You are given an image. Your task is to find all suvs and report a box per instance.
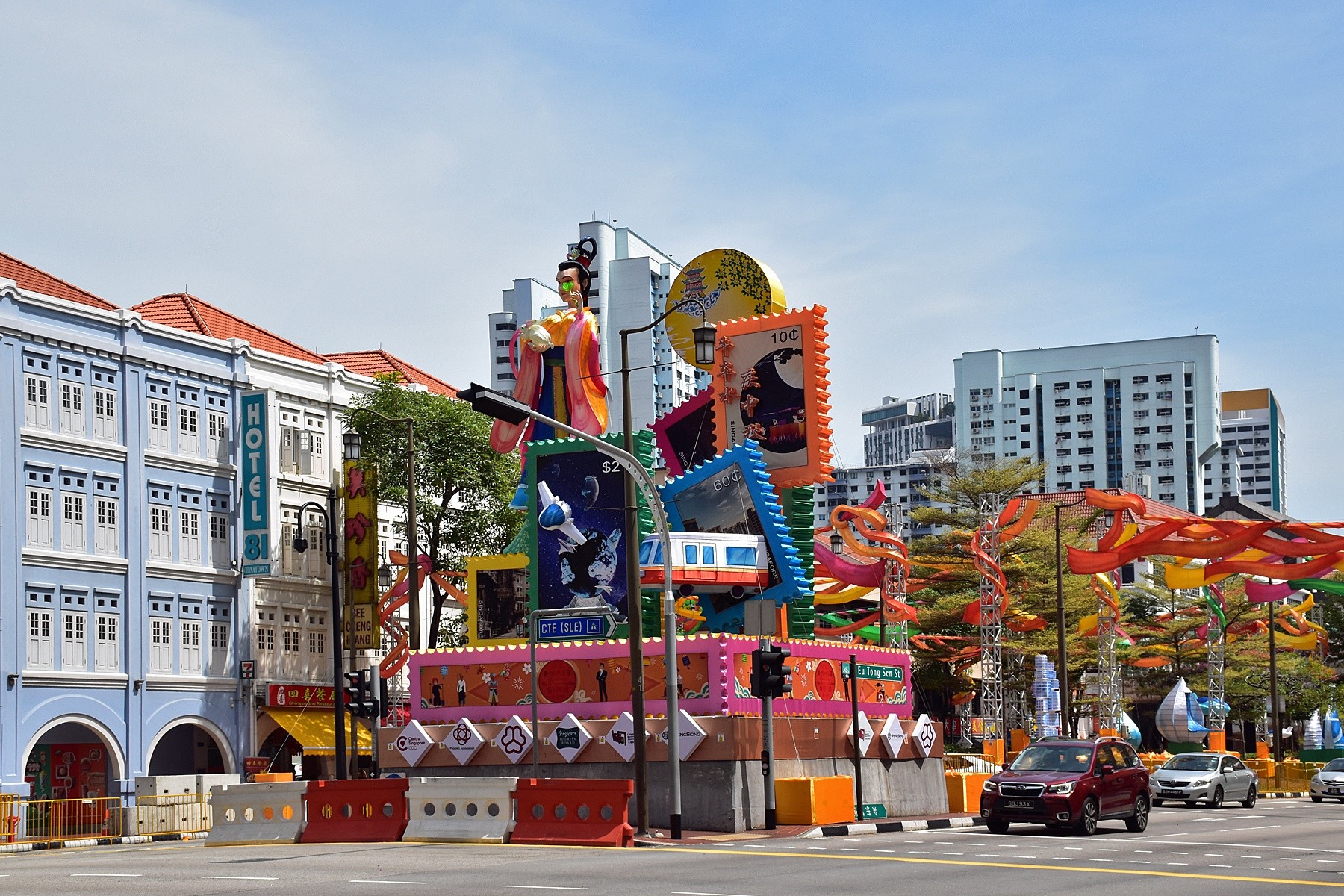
[979,734,1152,837]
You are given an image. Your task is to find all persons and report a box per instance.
[491,237,608,509]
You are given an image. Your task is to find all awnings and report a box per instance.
[380,705,411,727]
[259,706,373,756]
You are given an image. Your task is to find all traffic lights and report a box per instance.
[751,648,785,697]
[344,669,366,718]
[771,644,792,700]
[359,699,379,720]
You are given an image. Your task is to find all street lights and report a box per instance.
[1054,489,1115,740]
[617,298,719,835]
[292,500,348,780]
[456,381,684,840]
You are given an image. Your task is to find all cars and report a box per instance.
[1149,751,1259,809]
[942,752,1004,776]
[1309,758,1344,805]
